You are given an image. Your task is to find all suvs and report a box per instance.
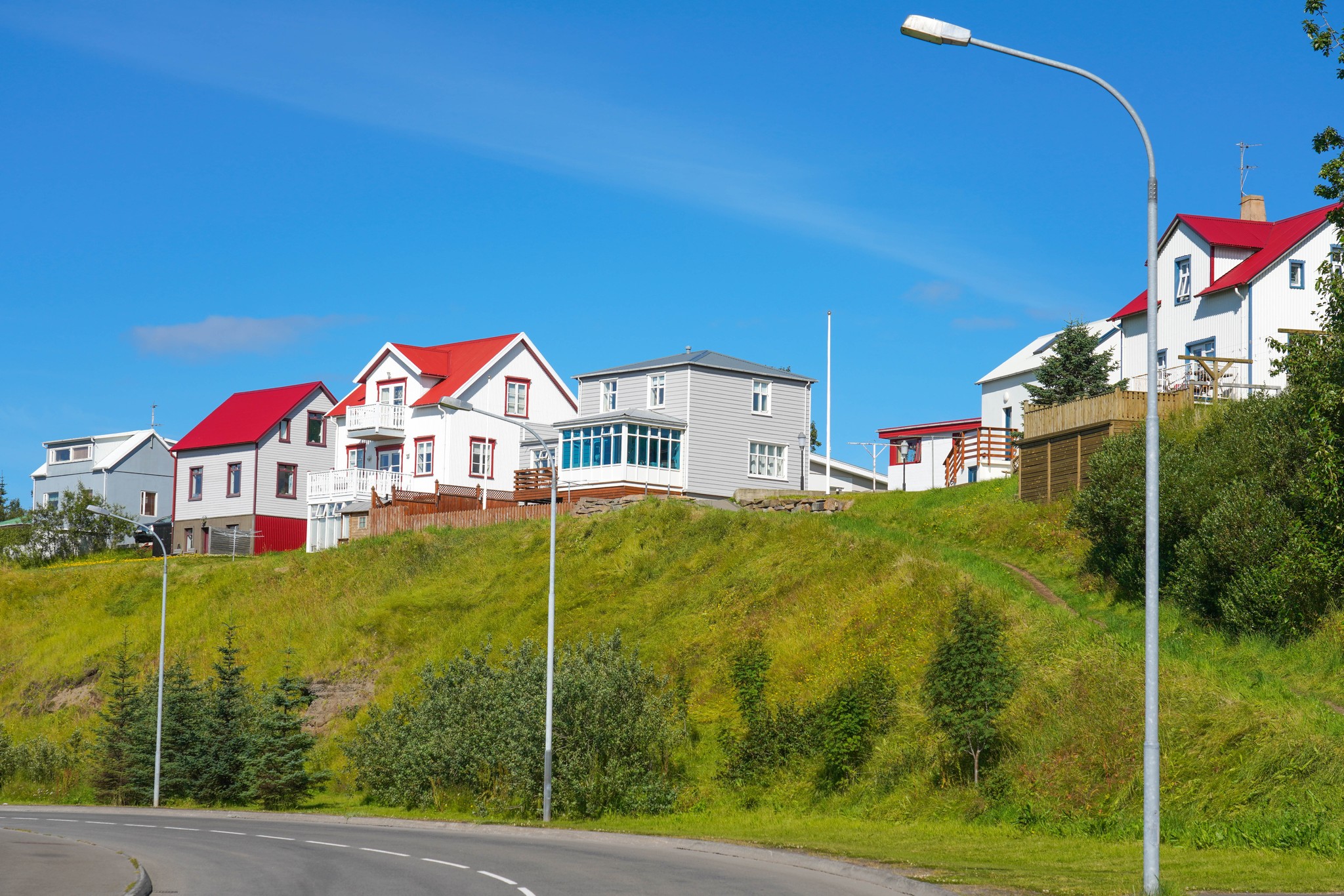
[133,514,173,543]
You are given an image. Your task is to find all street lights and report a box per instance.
[900,440,909,492]
[439,395,557,824]
[85,503,168,809]
[899,11,1160,896]
[797,432,806,490]
